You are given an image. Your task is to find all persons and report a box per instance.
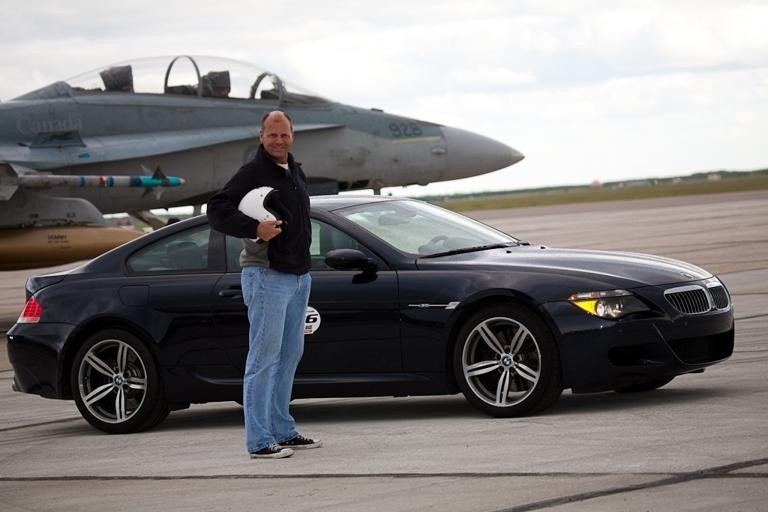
[207,111,323,458]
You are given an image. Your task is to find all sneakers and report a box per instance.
[277,433,325,449]
[249,440,294,459]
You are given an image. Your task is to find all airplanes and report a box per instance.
[0,49,535,276]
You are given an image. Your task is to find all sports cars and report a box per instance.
[2,193,738,441]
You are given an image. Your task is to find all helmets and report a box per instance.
[235,185,285,244]
